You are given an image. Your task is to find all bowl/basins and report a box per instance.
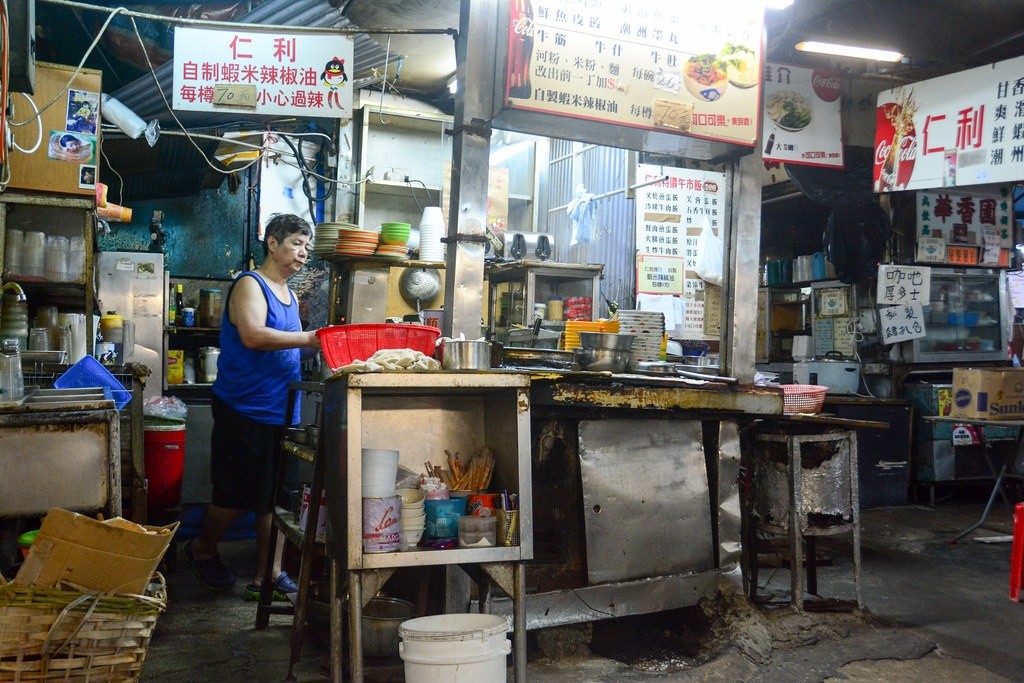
[634,361,679,375]
[380,207,447,263]
[766,91,813,133]
[396,486,426,546]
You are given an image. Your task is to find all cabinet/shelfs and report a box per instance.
[755,287,810,361]
[488,262,605,338]
[7,60,105,195]
[316,368,538,683]
[355,101,547,257]
[912,265,1007,365]
[743,427,866,612]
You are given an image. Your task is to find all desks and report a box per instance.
[919,414,1024,547]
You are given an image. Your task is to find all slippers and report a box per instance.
[181,537,235,590]
[244,570,298,601]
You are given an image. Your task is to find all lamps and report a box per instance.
[796,35,903,73]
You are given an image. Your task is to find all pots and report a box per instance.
[440,340,492,371]
[573,331,638,372]
[340,595,418,657]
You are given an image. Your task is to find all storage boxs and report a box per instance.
[814,319,833,359]
[832,318,853,356]
[820,287,849,317]
[948,365,1024,418]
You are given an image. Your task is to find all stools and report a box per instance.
[1008,501,1024,602]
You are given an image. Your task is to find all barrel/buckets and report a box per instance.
[142,424,187,509]
[360,495,402,554]
[398,612,512,683]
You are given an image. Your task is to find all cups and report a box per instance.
[101,314,135,365]
[180,307,196,326]
[425,488,502,547]
[0,230,85,282]
[198,288,222,328]
[71,322,98,363]
[28,328,48,352]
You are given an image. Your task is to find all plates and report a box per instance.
[565,308,667,364]
[313,222,410,257]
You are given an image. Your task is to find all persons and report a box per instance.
[71,100,90,131]
[180,214,322,601]
[84,170,93,184]
[60,135,80,153]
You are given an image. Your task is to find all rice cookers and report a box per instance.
[793,349,860,396]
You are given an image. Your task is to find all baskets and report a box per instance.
[315,323,441,370]
[56,354,132,411]
[778,385,830,413]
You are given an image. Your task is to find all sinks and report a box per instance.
[32,385,111,395]
[24,396,116,411]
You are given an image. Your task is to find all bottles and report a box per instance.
[764,130,776,155]
[878,131,903,192]
[168,282,184,325]
[199,346,220,382]
[0,337,24,402]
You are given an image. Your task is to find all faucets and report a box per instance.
[0,282,27,304]
